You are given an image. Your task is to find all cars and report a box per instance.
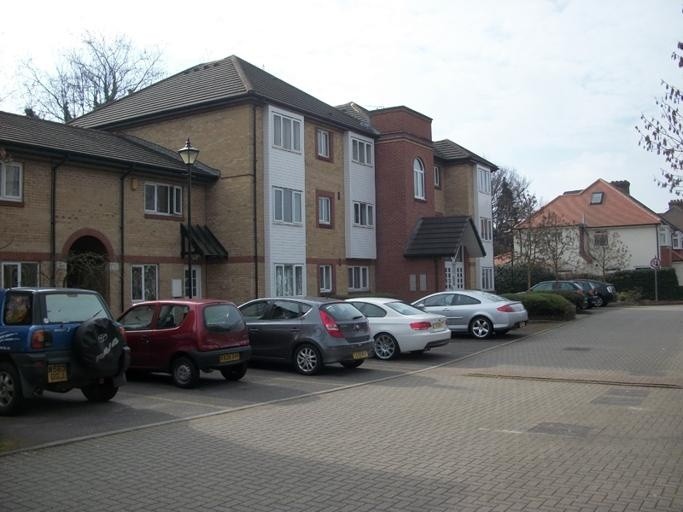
[516,281,598,313]
[335,294,456,368]
[224,294,376,379]
[405,288,530,342]
[105,295,257,393]
[567,278,617,308]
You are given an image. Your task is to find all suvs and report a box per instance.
[0,282,133,417]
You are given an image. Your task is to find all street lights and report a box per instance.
[176,135,201,302]
